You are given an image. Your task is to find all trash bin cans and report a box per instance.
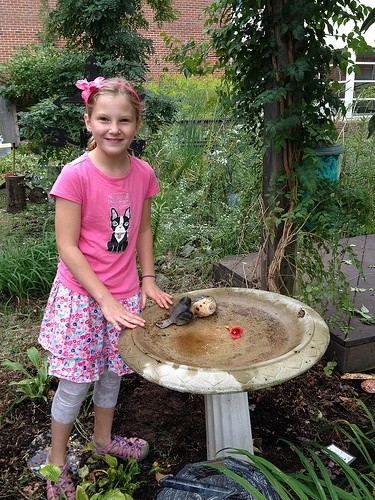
[302,140,344,233]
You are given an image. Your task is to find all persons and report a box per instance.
[36,75,174,498]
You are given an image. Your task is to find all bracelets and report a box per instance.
[142,275,156,280]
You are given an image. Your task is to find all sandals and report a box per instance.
[90,436,148,462]
[44,462,76,500]
